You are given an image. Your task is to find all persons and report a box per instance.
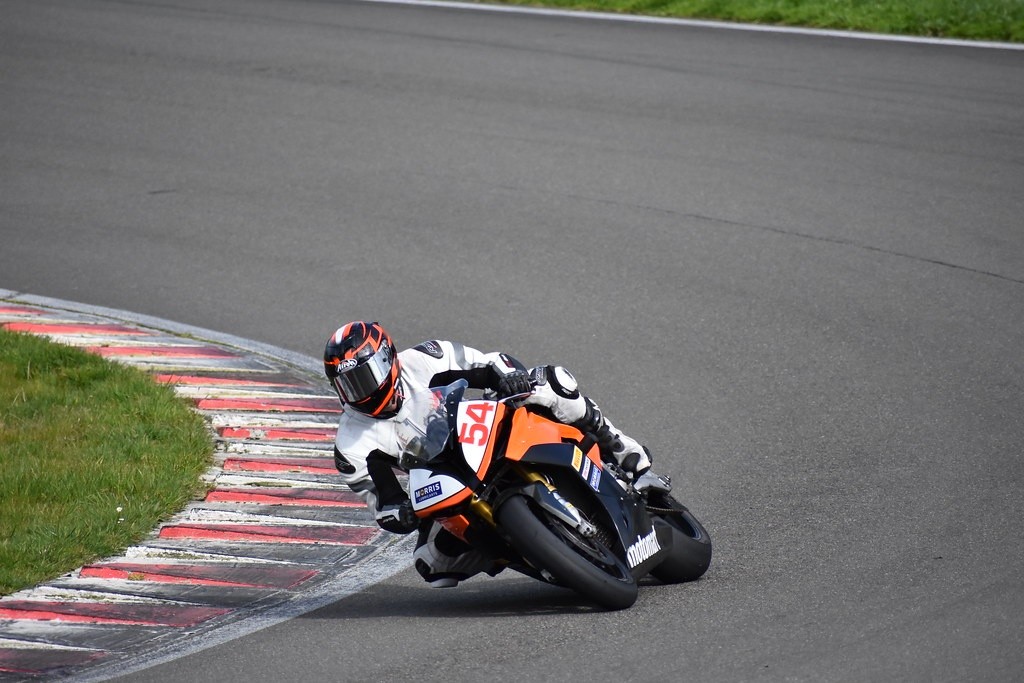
[325,321,652,589]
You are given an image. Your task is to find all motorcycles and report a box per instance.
[393,378,713,611]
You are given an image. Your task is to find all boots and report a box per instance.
[571,397,651,474]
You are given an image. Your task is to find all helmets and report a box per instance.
[323,320,401,417]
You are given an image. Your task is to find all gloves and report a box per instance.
[499,369,532,401]
[398,500,422,531]
